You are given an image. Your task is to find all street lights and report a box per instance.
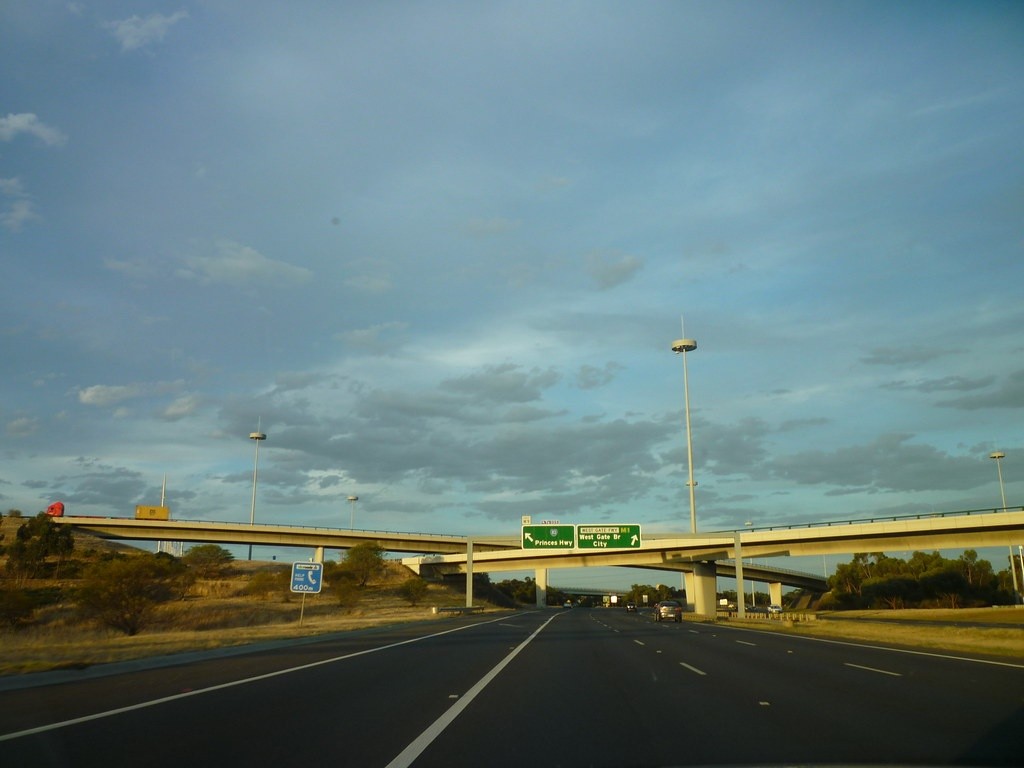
[989,447,1018,593]
[247,416,267,561]
[671,315,698,534]
[744,520,756,607]
[348,494,358,528]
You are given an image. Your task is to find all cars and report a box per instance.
[653,600,682,623]
[627,602,638,613]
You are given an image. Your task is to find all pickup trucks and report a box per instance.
[767,604,783,614]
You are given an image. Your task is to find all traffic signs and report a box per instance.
[575,524,642,548]
[521,524,576,551]
